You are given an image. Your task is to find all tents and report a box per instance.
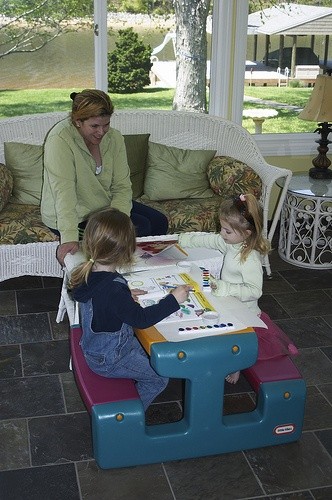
[245,2,332,76]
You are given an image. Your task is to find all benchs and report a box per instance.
[0,109,293,284]
[224,311,306,453]
[69,324,185,469]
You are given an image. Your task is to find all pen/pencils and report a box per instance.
[164,285,196,293]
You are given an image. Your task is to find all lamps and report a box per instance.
[297,74,332,179]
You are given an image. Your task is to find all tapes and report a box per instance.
[202,311,220,325]
[176,261,193,272]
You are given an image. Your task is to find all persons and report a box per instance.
[39,88,169,268]
[67,208,191,416]
[176,193,275,384]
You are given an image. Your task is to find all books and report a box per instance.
[127,271,217,325]
[131,240,179,259]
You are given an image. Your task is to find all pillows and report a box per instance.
[123,133,150,201]
[207,156,263,200]
[143,140,217,201]
[3,141,43,205]
[0,163,14,211]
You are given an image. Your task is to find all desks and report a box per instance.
[120,270,259,432]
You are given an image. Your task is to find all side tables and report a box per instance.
[275,170,332,270]
